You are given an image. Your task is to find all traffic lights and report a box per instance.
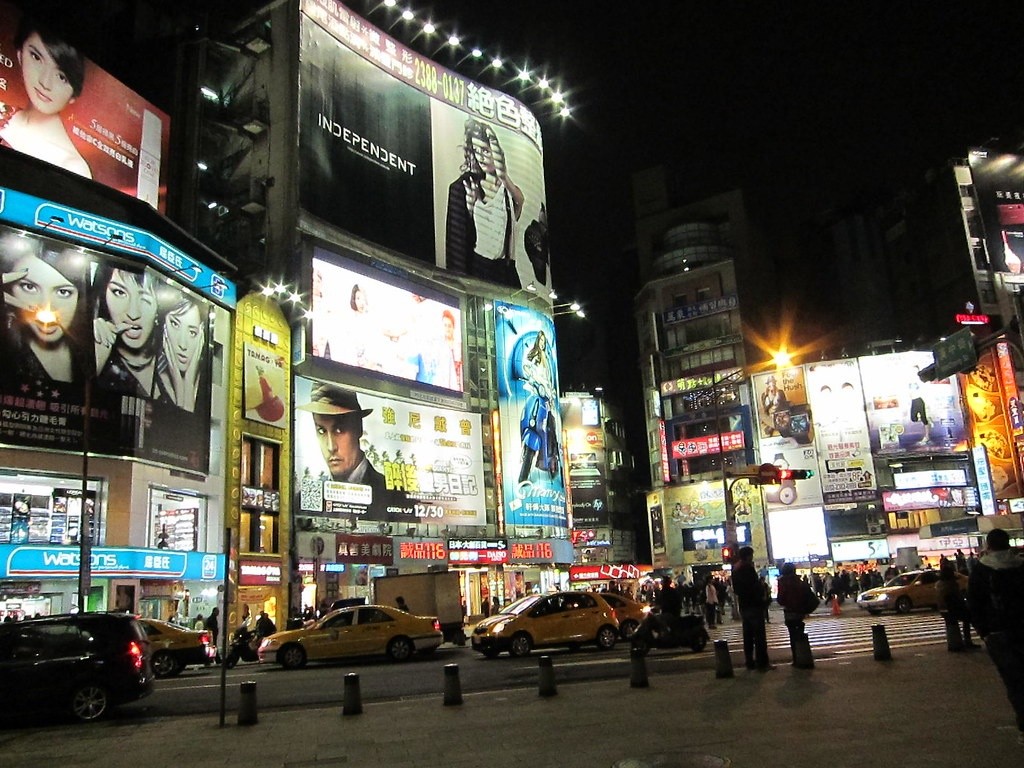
[722,545,735,564]
[781,468,813,480]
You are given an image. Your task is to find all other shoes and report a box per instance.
[708,625,717,629]
[1016,732,1024,744]
[745,660,773,672]
[964,639,982,649]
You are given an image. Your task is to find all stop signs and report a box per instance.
[758,462,777,484]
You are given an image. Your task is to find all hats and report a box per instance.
[295,382,373,418]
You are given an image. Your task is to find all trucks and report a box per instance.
[332,570,464,642]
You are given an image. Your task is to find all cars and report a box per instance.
[562,593,651,639]
[856,568,968,616]
[139,617,218,678]
[257,596,444,667]
[472,590,623,656]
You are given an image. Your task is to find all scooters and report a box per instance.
[224,626,260,668]
[518,365,560,482]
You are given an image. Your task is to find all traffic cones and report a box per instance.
[831,598,842,616]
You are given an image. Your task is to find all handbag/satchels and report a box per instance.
[802,583,820,614]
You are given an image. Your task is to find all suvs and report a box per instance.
[0,613,154,728]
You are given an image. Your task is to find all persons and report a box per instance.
[967,528,1024,732]
[195,603,330,665]
[153,281,210,415]
[0,11,92,180]
[731,546,770,671]
[908,364,935,446]
[445,115,525,289]
[914,548,982,652]
[294,381,421,523]
[761,375,786,435]
[461,596,500,627]
[89,263,158,397]
[312,266,464,392]
[526,330,557,473]
[0,227,89,405]
[396,596,409,613]
[776,562,815,669]
[592,565,900,630]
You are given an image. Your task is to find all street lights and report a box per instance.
[36,310,86,612]
[713,351,790,566]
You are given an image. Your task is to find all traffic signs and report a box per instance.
[931,325,979,381]
